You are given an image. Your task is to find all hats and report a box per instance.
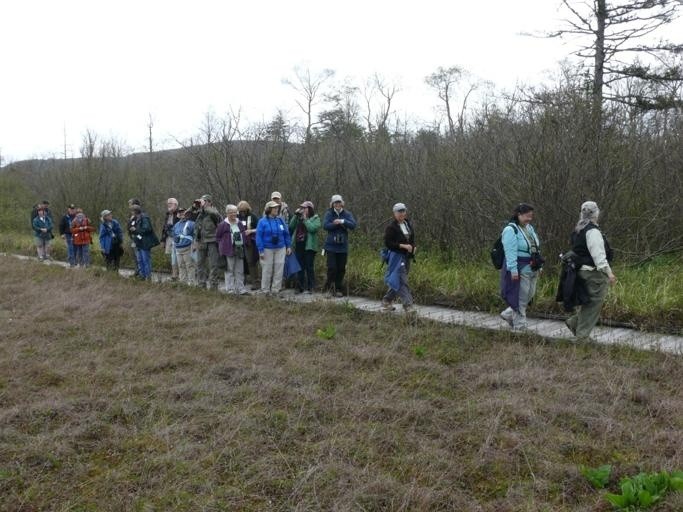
[393,203,406,211]
[101,210,112,216]
[330,195,344,207]
[201,194,211,202]
[300,201,313,208]
[265,192,281,208]
[581,201,597,214]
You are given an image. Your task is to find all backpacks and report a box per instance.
[491,224,518,269]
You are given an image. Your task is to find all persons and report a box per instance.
[380,202,418,315]
[500,203,546,332]
[320,195,356,300]
[556,200,617,343]
[31,200,96,269]
[128,191,322,296]
[97,210,124,275]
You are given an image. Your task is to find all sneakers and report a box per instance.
[403,305,417,313]
[228,289,247,295]
[383,303,395,311]
[500,311,529,332]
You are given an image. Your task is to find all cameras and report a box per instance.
[531,253,545,272]
[194,199,201,207]
[272,236,281,246]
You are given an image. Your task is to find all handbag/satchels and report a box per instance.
[137,217,160,249]
[381,247,390,263]
[285,252,301,278]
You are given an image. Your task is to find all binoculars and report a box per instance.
[337,234,344,244]
[193,200,202,204]
[532,252,542,268]
[294,208,305,215]
[234,232,241,241]
[406,244,415,258]
